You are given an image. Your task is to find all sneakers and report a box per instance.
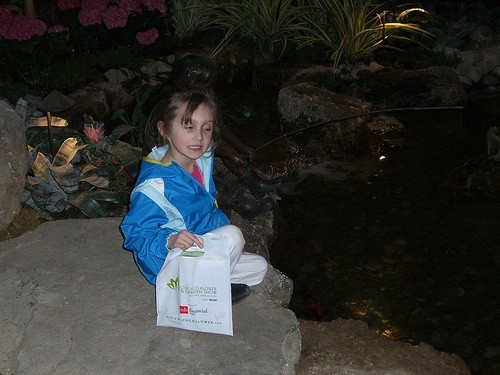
[229,283,250,305]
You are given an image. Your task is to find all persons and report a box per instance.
[119,82,269,308]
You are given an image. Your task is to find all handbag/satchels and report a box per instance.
[153,233,234,337]
